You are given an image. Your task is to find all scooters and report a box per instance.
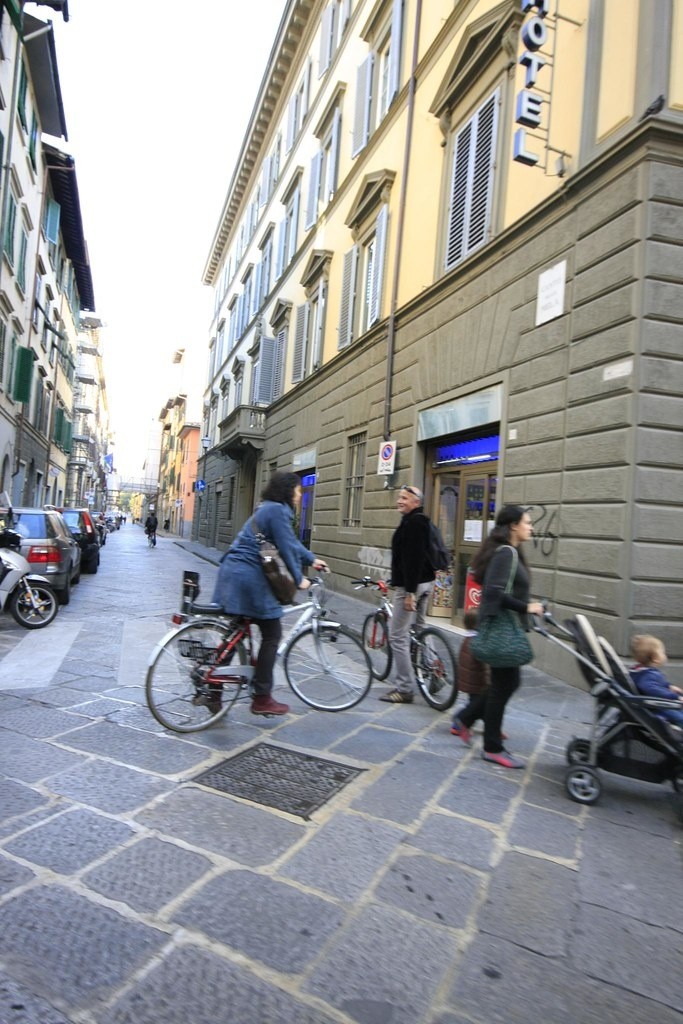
[0,490,59,630]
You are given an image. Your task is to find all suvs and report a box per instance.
[55,505,103,574]
[0,504,84,605]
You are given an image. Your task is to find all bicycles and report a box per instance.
[145,526,157,545]
[145,562,373,735]
[351,575,459,711]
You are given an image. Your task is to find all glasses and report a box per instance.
[401,485,420,498]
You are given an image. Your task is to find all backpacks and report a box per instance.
[409,512,452,572]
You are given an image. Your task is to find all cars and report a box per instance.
[89,510,124,545]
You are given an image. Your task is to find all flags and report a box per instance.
[104,454,113,472]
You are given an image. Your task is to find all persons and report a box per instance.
[200,470,332,717]
[453,496,538,774]
[143,511,157,546]
[382,486,452,704]
[627,628,682,736]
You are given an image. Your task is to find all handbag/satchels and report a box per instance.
[468,601,533,668]
[256,534,295,605]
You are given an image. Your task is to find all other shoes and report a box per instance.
[450,726,474,735]
[454,719,475,746]
[482,748,527,768]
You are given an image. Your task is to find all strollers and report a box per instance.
[527,597,682,805]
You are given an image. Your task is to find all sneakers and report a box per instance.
[206,690,222,713]
[426,672,445,695]
[251,692,289,715]
[380,689,414,703]
